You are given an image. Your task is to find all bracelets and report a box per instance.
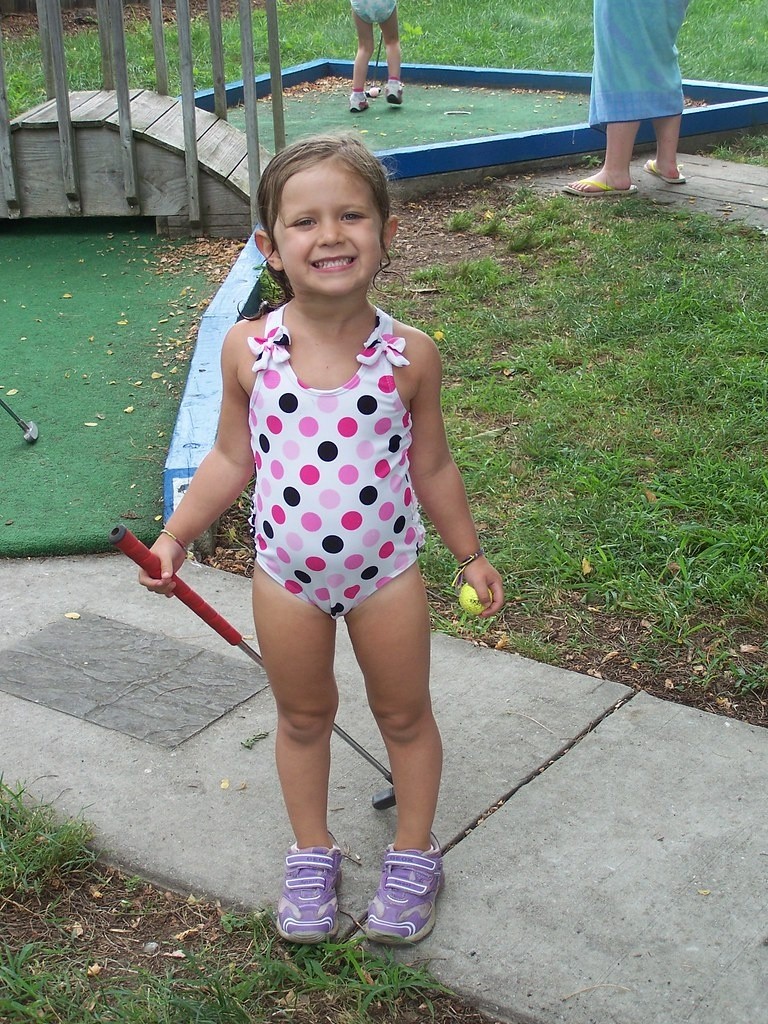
[451,547,484,588]
[160,530,188,558]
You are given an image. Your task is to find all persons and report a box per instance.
[564,0,691,196]
[350,0,403,111]
[137,133,506,947]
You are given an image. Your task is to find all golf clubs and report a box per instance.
[108,523,397,811]
[365,31,382,97]
[0,399,39,443]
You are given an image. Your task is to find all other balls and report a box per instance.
[369,88,378,97]
[457,583,493,615]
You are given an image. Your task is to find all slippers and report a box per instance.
[643,157,686,185]
[562,178,639,196]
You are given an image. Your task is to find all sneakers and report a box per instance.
[384,83,402,105]
[277,829,343,943]
[365,830,444,945]
[349,94,369,112]
